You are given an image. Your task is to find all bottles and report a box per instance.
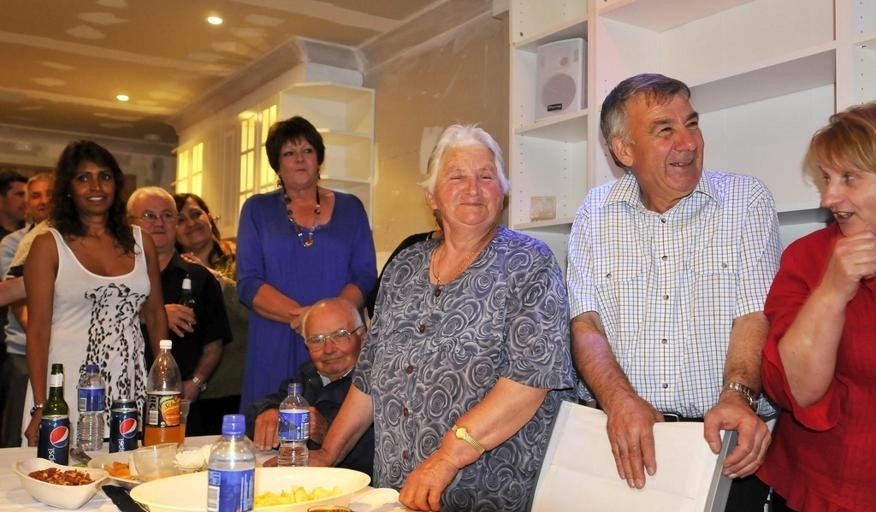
[277,383,310,467]
[205,414,255,512]
[144,340,181,449]
[76,365,104,451]
[177,272,195,330]
[42,364,68,415]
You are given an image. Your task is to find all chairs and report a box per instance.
[527,397,738,511]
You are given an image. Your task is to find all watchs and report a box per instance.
[720,382,761,411]
[450,422,488,458]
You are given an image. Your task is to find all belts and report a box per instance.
[662,414,704,422]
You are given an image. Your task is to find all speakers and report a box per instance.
[534,37,586,122]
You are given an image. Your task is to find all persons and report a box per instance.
[563,72,783,512]
[243,297,375,486]
[234,115,377,436]
[18,138,181,446]
[259,116,582,512]
[363,140,442,323]
[753,98,876,511]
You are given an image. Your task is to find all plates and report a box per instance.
[86,450,141,489]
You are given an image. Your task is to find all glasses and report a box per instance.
[129,212,177,222]
[302,327,361,348]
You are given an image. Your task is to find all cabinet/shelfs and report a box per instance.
[508,0,876,279]
[278,79,377,234]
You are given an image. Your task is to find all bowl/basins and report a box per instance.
[129,466,371,512]
[13,456,108,509]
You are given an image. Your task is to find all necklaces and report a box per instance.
[430,227,493,286]
[281,186,319,248]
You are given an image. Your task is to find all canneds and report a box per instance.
[109,408,137,453]
[38,414,69,465]
[111,399,137,408]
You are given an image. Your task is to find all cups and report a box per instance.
[132,442,176,482]
[529,195,556,221]
[177,398,190,443]
[306,505,352,512]
